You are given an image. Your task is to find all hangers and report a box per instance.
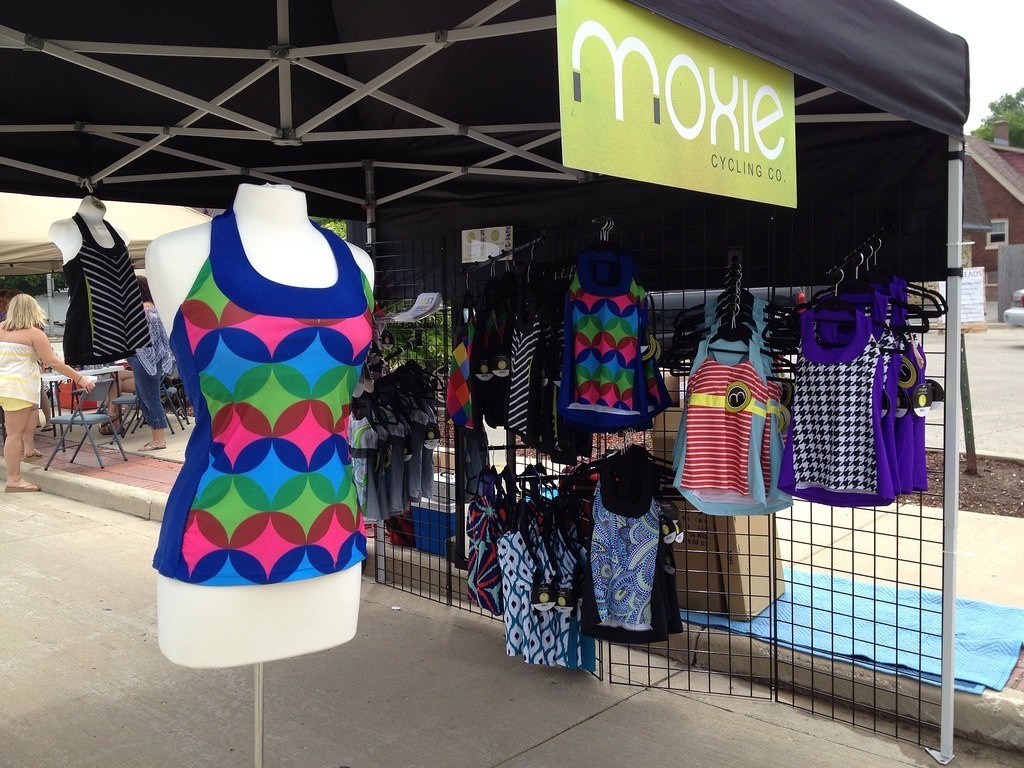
[658,232,949,383]
[451,427,687,576]
[352,340,443,427]
[445,212,614,292]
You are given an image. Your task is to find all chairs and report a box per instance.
[110,358,190,446]
[44,378,128,471]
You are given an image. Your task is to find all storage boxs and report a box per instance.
[411,498,456,555]
[58,376,97,409]
[650,368,785,621]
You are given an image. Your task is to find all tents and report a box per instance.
[0,192,216,276]
[0,0,973,764]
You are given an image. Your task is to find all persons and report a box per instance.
[98,362,178,436]
[145,183,376,670]
[49,195,152,364]
[0,288,97,493]
[126,275,174,452]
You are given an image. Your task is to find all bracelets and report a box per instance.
[77,376,82,383]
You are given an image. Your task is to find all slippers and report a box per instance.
[4,481,39,493]
[98,425,123,435]
[24,449,43,462]
[36,426,41,428]
[41,424,53,432]
[138,440,166,451]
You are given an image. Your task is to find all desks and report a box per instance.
[41,366,126,451]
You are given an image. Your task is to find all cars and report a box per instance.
[648,286,807,364]
[374,299,477,406]
